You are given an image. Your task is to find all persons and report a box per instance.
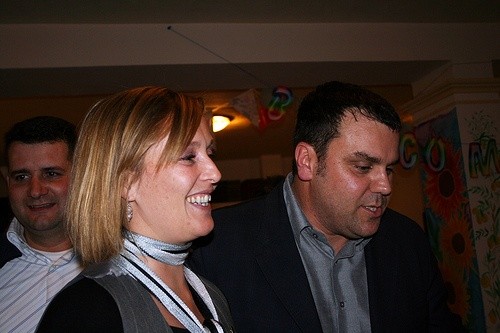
[34,84,238,333]
[0,114,87,333]
[183,79,471,333]
